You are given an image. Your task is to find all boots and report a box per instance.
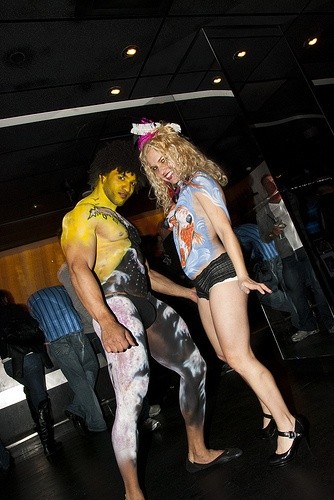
[35,399,63,456]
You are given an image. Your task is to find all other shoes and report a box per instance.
[64,409,86,438]
[223,364,234,373]
[186,447,242,473]
[143,418,162,431]
[291,328,320,342]
[149,404,162,417]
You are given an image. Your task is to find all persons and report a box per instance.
[257,174,334,342]
[0,290,55,455]
[130,118,310,470]
[233,224,317,342]
[60,142,243,500]
[57,262,107,362]
[27,286,107,431]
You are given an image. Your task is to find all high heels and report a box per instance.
[256,414,277,441]
[267,416,311,465]
